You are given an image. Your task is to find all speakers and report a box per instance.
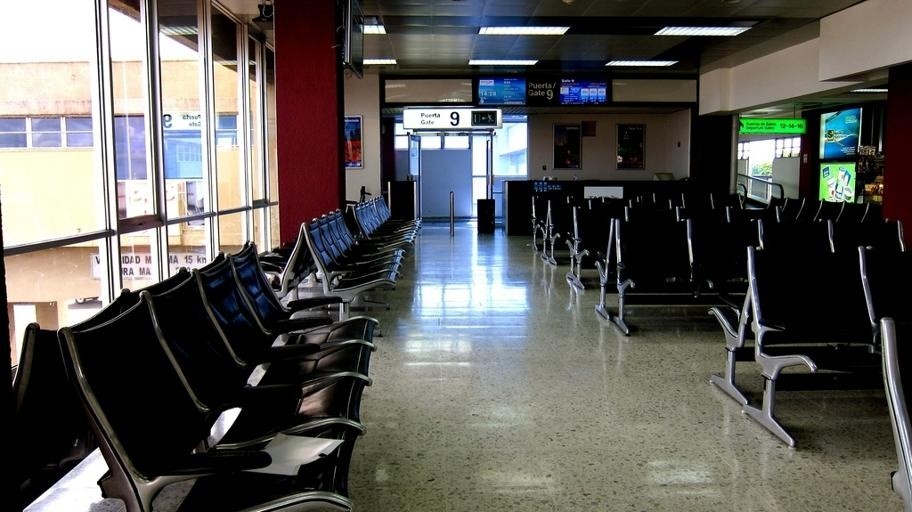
[477,199,495,234]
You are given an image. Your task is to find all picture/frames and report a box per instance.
[344,114,364,170]
[552,122,582,170]
[615,124,646,170]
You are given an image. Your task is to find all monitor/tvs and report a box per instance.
[344,0,364,80]
[471,76,527,108]
[557,75,613,107]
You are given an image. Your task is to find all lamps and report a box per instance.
[467,26,571,66]
[601,21,759,67]
[357,14,398,65]
